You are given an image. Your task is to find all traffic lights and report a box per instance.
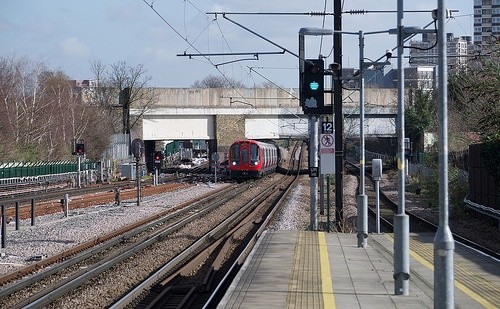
[304,58,324,113]
[76,143,84,155]
[154,151,161,168]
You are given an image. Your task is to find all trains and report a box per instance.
[228,139,278,182]
